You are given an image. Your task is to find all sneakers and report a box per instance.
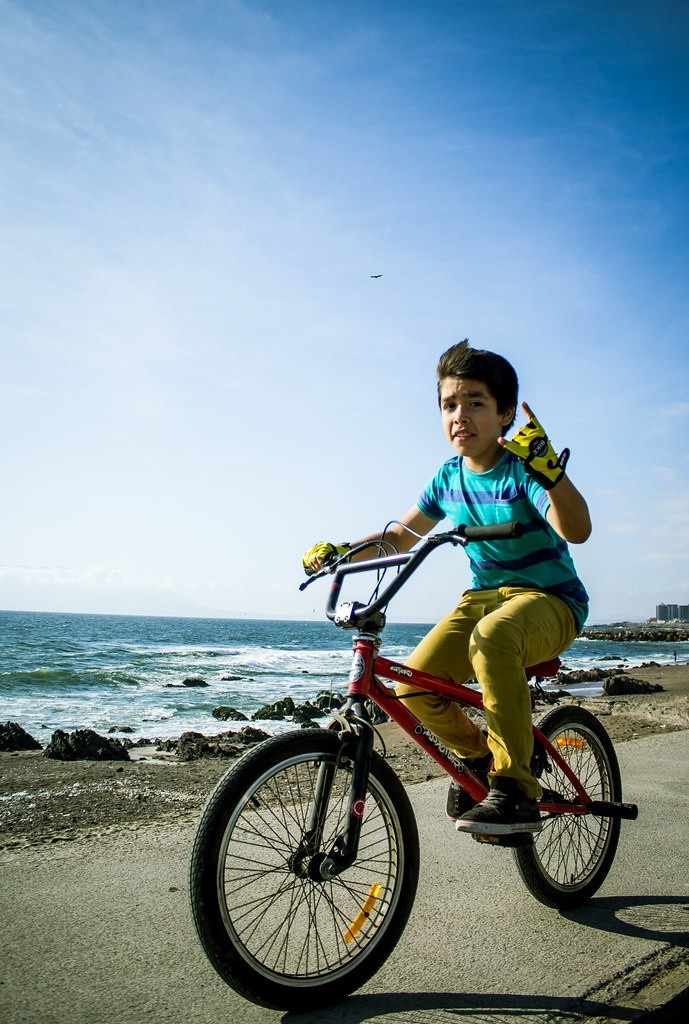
[444,729,494,822]
[456,777,542,834]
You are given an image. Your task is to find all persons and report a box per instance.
[299,340,592,829]
[671,649,677,663]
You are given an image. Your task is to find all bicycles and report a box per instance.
[186,516,640,1017]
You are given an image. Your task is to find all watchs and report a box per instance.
[337,541,353,563]
